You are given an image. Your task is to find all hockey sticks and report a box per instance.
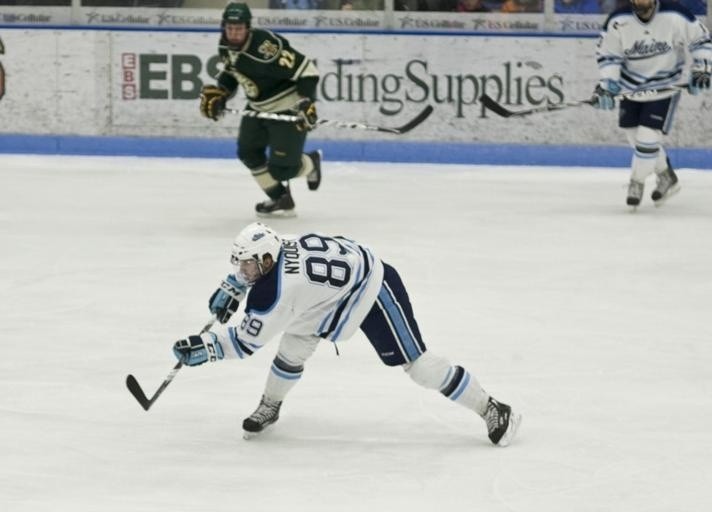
[223,105,433,134]
[479,85,689,118]
[127,314,219,411]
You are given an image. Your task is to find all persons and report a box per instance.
[175,223,512,444]
[588,0,691,204]
[673,1,712,94]
[200,3,324,214]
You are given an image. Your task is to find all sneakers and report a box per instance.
[650,166,679,200]
[307,150,321,191]
[242,392,283,432]
[255,189,295,214]
[479,395,512,445]
[625,178,644,206]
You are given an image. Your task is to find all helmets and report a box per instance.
[229,222,282,285]
[222,2,252,29]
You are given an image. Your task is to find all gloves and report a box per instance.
[171,326,245,367]
[199,85,227,120]
[590,78,623,111]
[687,70,712,96]
[294,98,318,133]
[208,273,248,325]
